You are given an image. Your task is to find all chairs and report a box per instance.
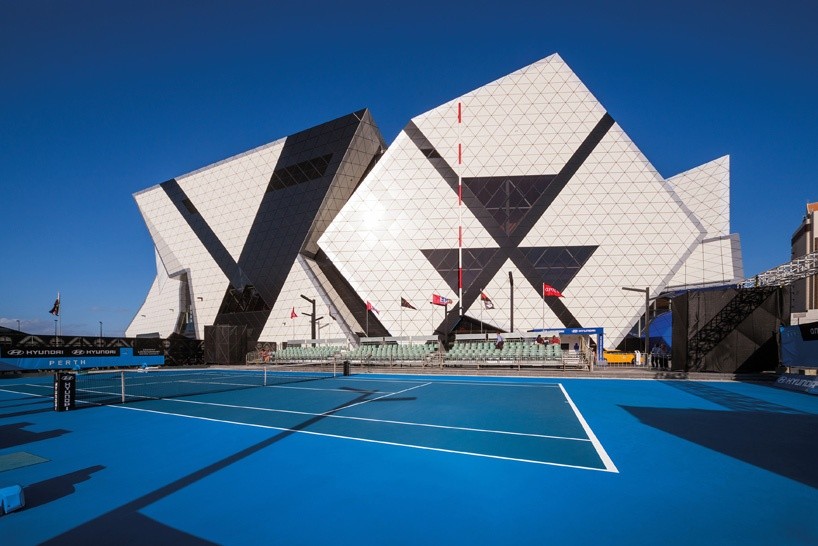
[272,339,564,360]
[562,359,583,369]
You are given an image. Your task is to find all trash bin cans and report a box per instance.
[343,361,350,376]
[53,372,76,412]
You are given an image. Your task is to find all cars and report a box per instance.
[604,350,645,364]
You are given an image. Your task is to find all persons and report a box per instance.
[535,335,579,353]
[650,344,669,368]
[494,332,503,350]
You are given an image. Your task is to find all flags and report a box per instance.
[401,297,418,311]
[481,292,494,310]
[543,283,565,297]
[367,301,379,315]
[433,295,453,306]
[290,308,298,319]
[50,300,59,315]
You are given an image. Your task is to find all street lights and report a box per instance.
[300,294,317,349]
[509,270,514,332]
[16,319,21,332]
[623,286,652,365]
[99,321,105,337]
